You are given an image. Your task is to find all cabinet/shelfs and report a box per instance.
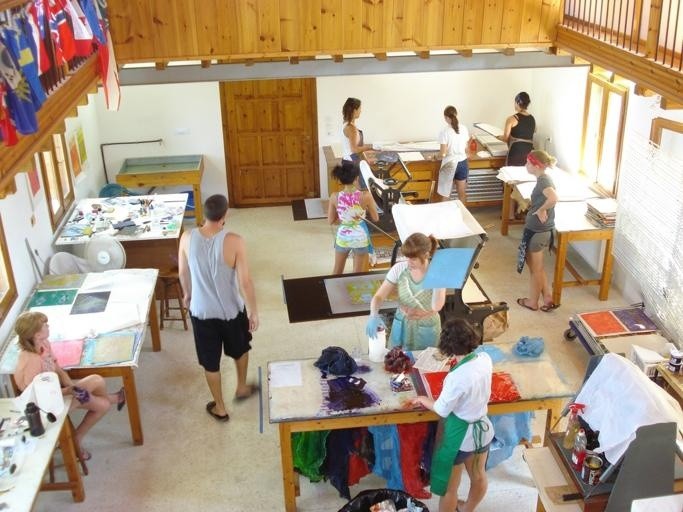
[534,431,683,512]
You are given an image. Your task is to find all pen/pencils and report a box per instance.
[163,200,184,202]
[139,198,153,215]
[8,428,29,436]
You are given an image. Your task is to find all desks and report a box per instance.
[265,348,574,512]
[321,139,508,208]
[500,171,616,306]
[652,358,683,405]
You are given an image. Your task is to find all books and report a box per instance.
[584,197,618,230]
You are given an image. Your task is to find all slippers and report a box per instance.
[57,442,91,460]
[541,301,561,311]
[117,389,127,411]
[236,383,259,400]
[517,297,537,311]
[207,401,229,423]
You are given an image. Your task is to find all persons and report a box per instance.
[432,106,470,209]
[176,193,260,424]
[340,97,375,193]
[406,316,494,510]
[516,148,560,313]
[364,232,448,350]
[326,160,380,278]
[494,92,537,221]
[12,311,127,462]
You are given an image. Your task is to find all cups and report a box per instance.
[580,455,604,485]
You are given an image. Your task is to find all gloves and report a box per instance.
[365,317,387,340]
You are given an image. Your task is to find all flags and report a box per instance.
[0,0,124,149]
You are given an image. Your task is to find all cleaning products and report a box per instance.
[470,135,477,151]
[563,403,585,449]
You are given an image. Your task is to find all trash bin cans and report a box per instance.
[336,488,430,512]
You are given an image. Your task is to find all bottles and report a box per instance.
[585,430,601,458]
[668,350,683,374]
[368,328,386,363]
[24,401,57,436]
